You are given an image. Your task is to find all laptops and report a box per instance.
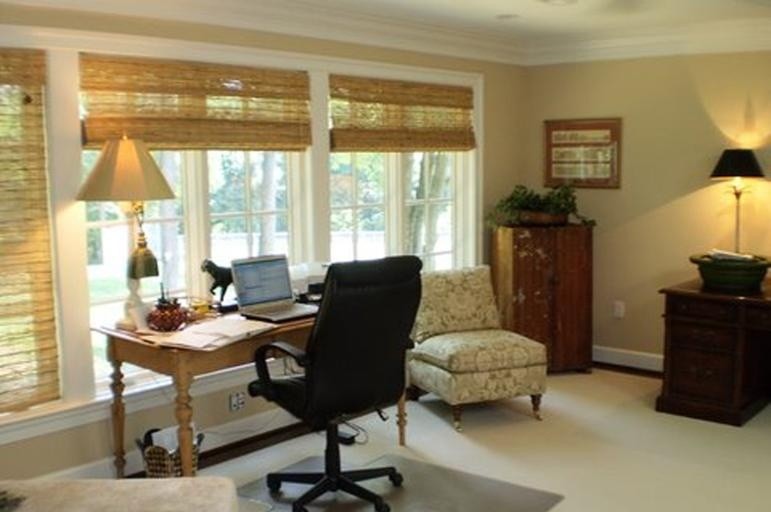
[231,254,319,324]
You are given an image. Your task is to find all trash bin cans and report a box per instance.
[136,428,205,478]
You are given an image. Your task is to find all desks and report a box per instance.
[90,298,407,478]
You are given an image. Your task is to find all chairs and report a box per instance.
[249,256,423,511]
[405,263,549,432]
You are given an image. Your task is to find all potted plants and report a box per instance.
[495,183,577,224]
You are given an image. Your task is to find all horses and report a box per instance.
[200,259,235,304]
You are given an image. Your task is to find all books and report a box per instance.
[707,247,759,262]
[128,301,279,353]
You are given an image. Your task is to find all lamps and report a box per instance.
[76,128,176,331]
[708,148,764,254]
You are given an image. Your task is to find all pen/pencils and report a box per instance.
[159,282,178,305]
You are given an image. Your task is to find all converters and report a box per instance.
[338,431,355,445]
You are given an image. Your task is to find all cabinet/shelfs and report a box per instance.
[656,275,771,427]
[492,222,593,373]
